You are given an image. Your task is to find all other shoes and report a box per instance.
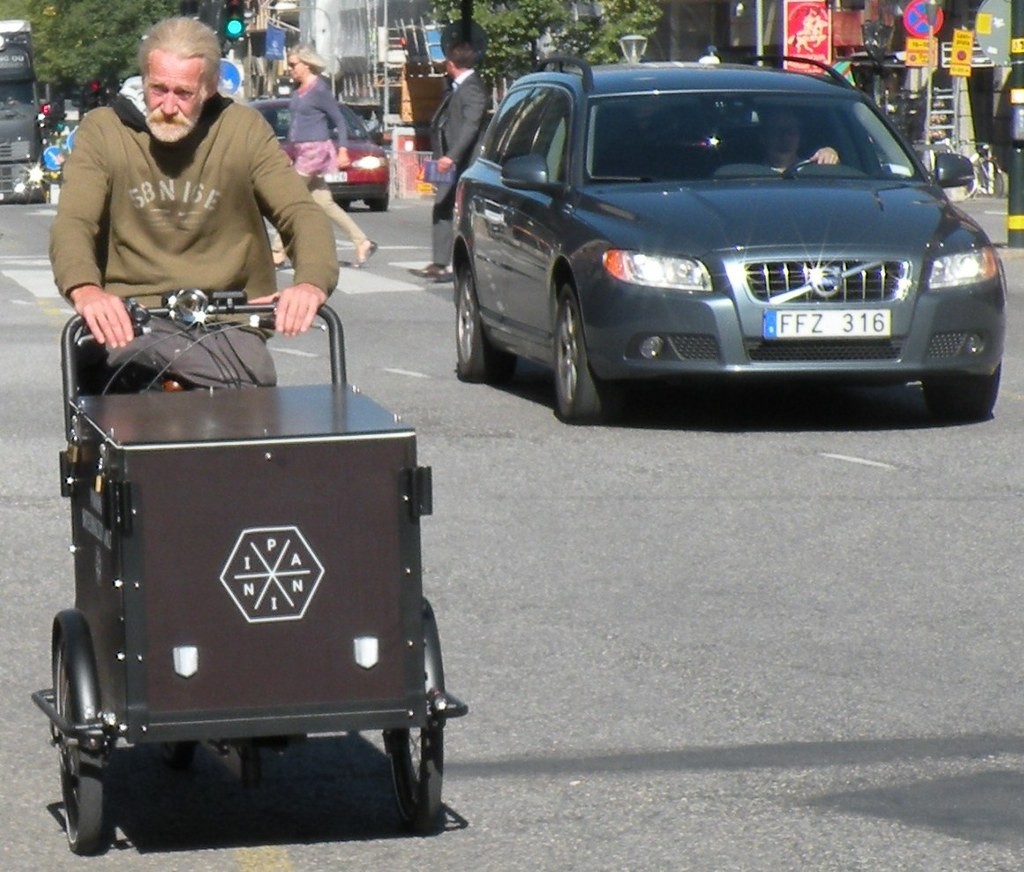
[345,241,378,270]
[272,260,287,272]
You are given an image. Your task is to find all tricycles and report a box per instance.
[30,282,469,859]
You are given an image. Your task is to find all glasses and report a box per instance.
[287,60,301,67]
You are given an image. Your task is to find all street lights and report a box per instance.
[276,2,338,96]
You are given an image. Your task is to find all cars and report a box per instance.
[248,96,394,213]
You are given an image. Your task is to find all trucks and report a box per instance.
[0,18,56,204]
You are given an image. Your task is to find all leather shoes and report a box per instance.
[407,264,452,282]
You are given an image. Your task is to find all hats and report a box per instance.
[296,45,327,73]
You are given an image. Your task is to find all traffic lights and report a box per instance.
[222,0,248,39]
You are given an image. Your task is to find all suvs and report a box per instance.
[450,53,1007,426]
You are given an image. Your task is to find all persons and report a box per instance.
[407,41,488,281]
[50,18,343,385]
[717,109,839,174]
[266,45,378,269]
